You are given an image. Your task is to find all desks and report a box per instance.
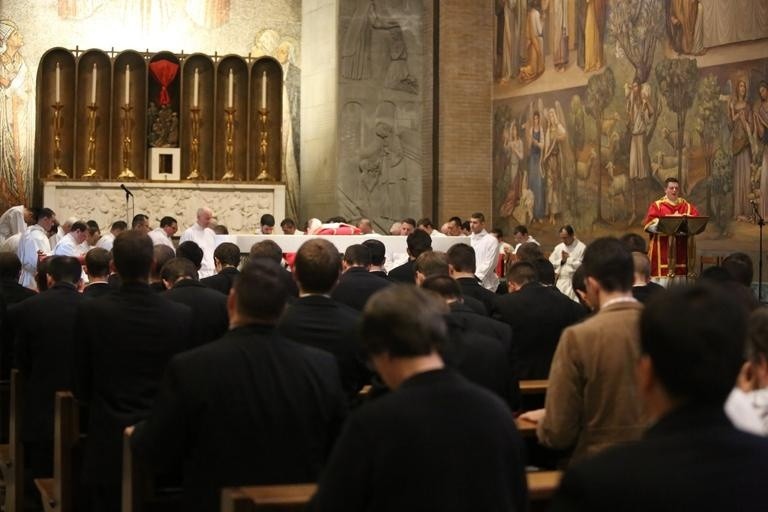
[220,234,475,288]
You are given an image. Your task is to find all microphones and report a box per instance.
[121,184,133,197]
[750,200,765,227]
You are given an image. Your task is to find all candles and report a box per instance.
[92,64,96,106]
[55,61,60,106]
[261,74,266,109]
[125,65,130,105]
[227,71,234,109]
[193,67,199,107]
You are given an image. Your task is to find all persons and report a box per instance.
[128,256,351,510]
[641,177,701,291]
[0,203,768,466]
[68,232,198,508]
[722,303,765,442]
[522,234,675,473]
[316,284,535,508]
[548,282,764,508]
